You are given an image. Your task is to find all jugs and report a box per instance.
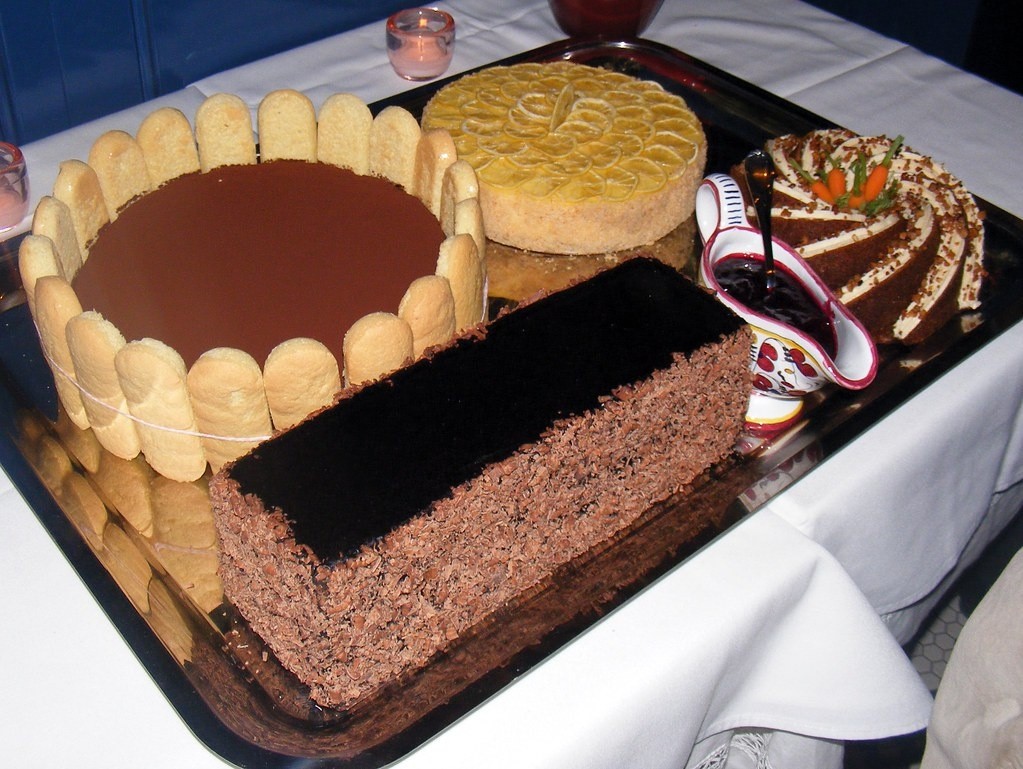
[697,173,878,430]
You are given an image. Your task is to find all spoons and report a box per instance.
[744,150,805,328]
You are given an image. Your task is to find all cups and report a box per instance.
[549,0,665,40]
[0,142,28,231]
[386,8,455,81]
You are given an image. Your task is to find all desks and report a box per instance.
[0,1,1023,769]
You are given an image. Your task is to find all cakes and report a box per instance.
[19,59,986,708]
